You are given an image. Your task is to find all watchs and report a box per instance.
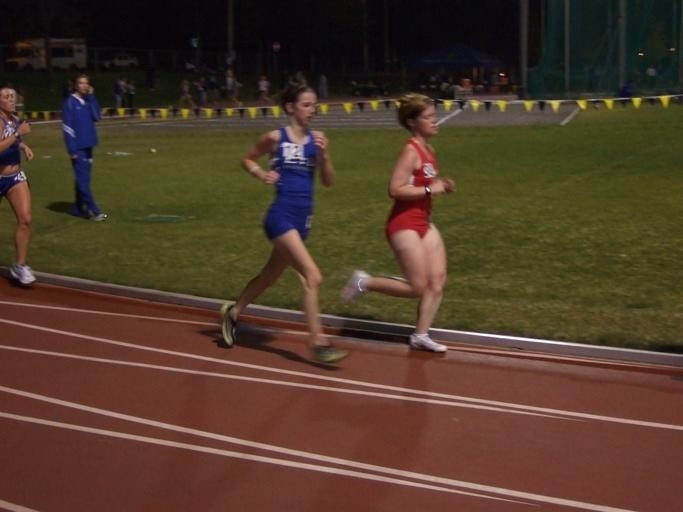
[425,184,432,196]
[12,130,19,139]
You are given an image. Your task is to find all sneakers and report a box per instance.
[221,301,236,345]
[340,271,369,307]
[11,264,37,284]
[410,332,447,352]
[310,343,348,362]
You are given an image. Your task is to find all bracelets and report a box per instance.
[248,166,260,175]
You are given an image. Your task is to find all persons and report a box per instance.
[1,84,38,286]
[218,82,352,366]
[340,90,456,355]
[61,74,107,222]
[106,69,638,113]
